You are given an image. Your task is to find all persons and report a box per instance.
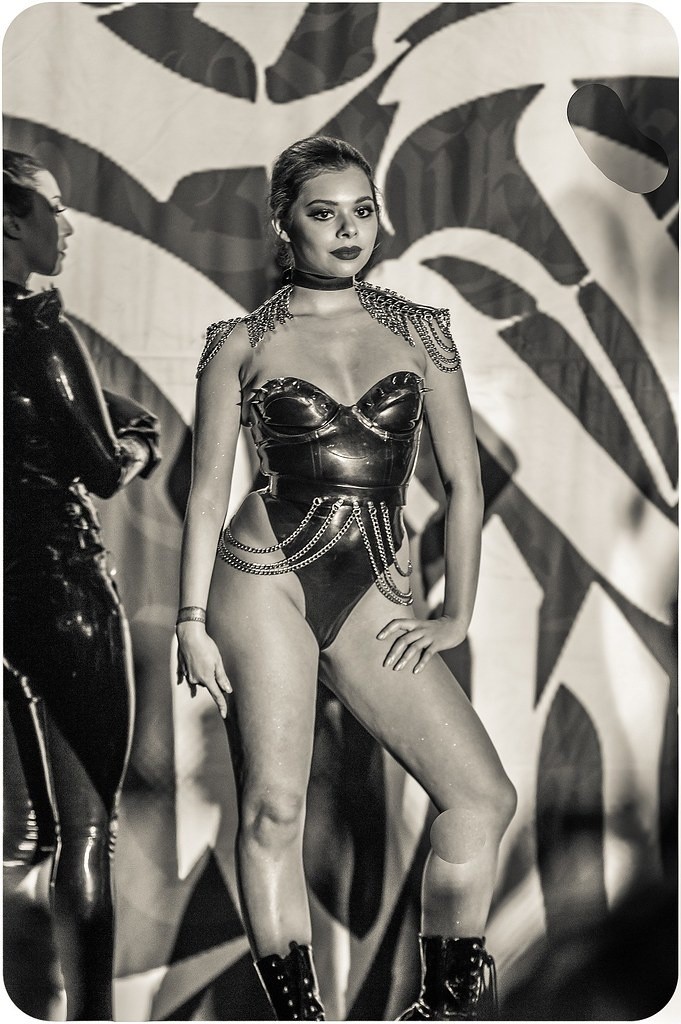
[175,134,518,1021]
[2,148,163,1021]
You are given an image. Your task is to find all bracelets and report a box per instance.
[176,607,208,626]
[118,415,162,481]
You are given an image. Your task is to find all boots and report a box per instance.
[393,934,498,1021]
[253,941,325,1021]
